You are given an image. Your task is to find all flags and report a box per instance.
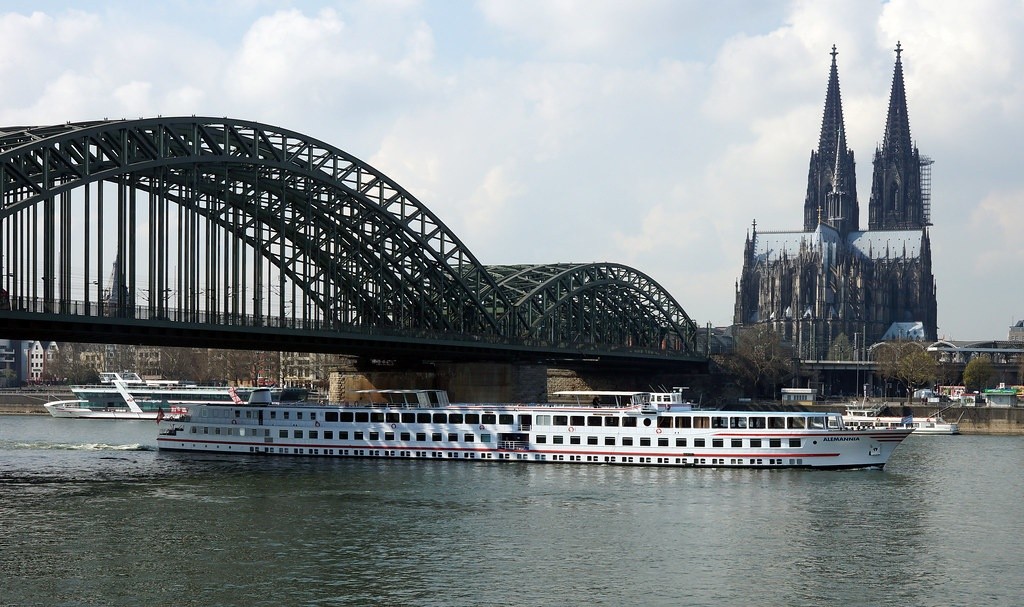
[901,415,913,425]
[156,407,164,425]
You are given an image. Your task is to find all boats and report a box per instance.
[43,369,284,421]
[154,381,918,473]
[828,406,961,436]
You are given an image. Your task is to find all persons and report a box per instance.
[593,396,601,408]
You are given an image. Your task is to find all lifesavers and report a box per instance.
[877,418,880,421]
[231,419,236,424]
[568,427,574,432]
[391,424,396,429]
[315,422,320,427]
[927,419,930,422]
[656,429,662,433]
[478,425,485,430]
